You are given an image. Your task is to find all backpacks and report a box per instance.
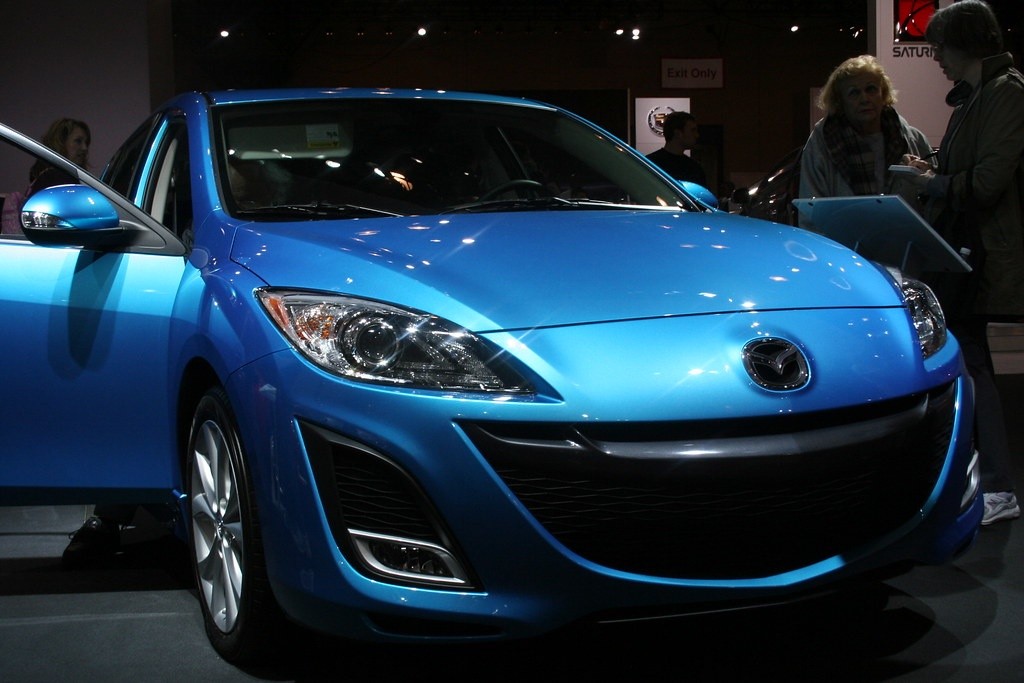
[1,167,52,234]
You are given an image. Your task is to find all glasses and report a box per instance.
[930,46,942,54]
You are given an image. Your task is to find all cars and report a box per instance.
[0,87,985,666]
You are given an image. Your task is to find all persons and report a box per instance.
[795,51,936,229]
[900,0,1024,525]
[644,112,708,188]
[29,116,92,236]
[59,503,139,566]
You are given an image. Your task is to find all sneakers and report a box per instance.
[981,491,1021,525]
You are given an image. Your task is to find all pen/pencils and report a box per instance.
[920,150,939,160]
